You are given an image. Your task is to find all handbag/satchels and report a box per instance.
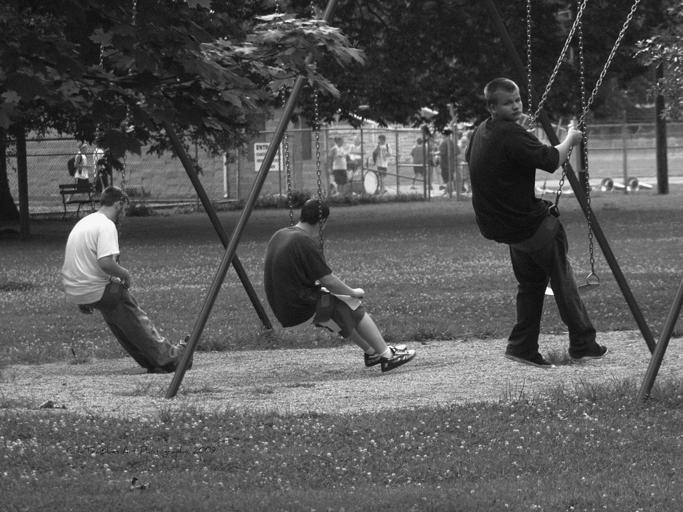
[67,154,82,176]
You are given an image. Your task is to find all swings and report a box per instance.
[505,1,637,254]
[74,2,136,315]
[524,2,601,298]
[274,1,333,324]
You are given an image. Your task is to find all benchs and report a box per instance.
[59,183,100,220]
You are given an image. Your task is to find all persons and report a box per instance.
[264,200,415,373]
[465,76,608,368]
[61,188,193,374]
[74,141,108,192]
[327,123,471,193]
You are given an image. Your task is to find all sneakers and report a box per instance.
[380,349,416,372]
[154,332,194,374]
[568,346,609,360]
[505,347,551,368]
[364,345,408,367]
